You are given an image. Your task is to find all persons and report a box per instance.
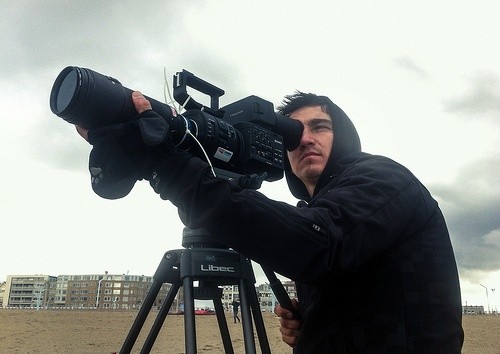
[73,90,464,354]
[232,300,240,323]
